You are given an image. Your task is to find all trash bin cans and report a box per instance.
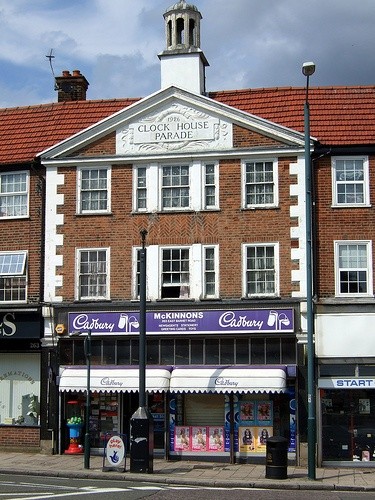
[264,436,290,479]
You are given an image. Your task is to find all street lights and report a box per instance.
[130,227,153,472]
[301,60,317,479]
[69,328,92,469]
